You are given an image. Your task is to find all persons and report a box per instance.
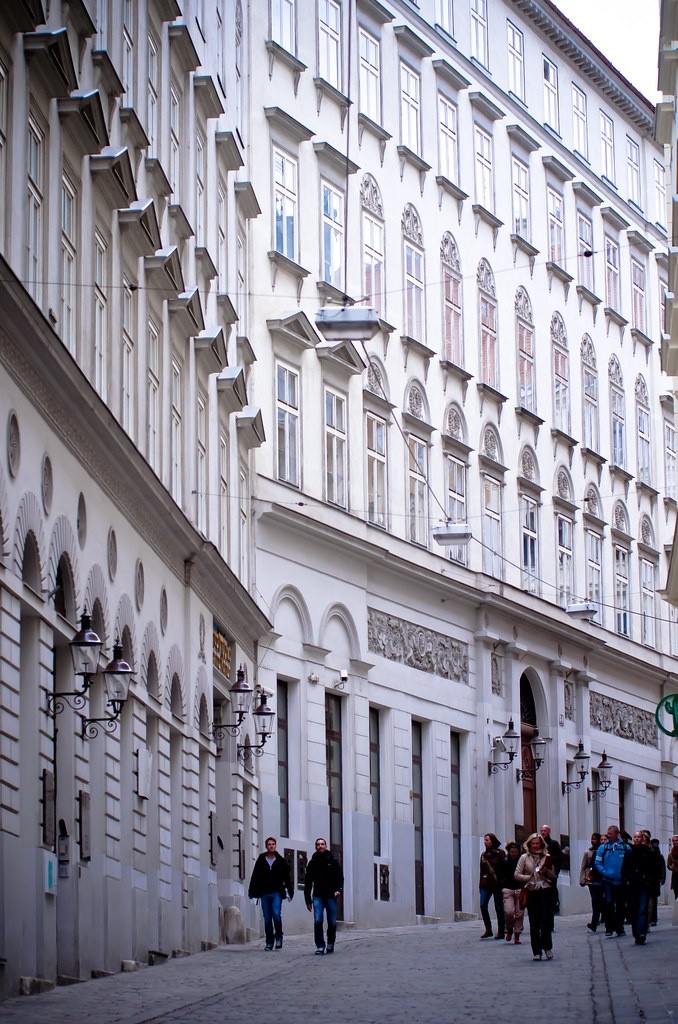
[247,837,295,951]
[579,826,678,945]
[304,838,344,954]
[479,825,561,961]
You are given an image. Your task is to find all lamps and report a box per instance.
[487,719,518,774]
[81,636,138,738]
[432,525,474,548]
[314,307,381,341]
[237,690,276,761]
[209,664,255,742]
[585,750,613,803]
[566,602,599,622]
[44,608,105,717]
[516,729,544,782]
[560,741,590,795]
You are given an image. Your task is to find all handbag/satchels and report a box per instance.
[519,887,527,910]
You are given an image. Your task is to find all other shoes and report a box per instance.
[635,934,646,946]
[605,931,613,936]
[481,931,493,938]
[264,944,274,951]
[326,944,334,953]
[586,923,596,932]
[533,955,542,961]
[506,931,513,941]
[617,931,626,936]
[276,940,282,949]
[315,947,324,955]
[514,935,522,944]
[495,933,505,939]
[545,951,553,959]
